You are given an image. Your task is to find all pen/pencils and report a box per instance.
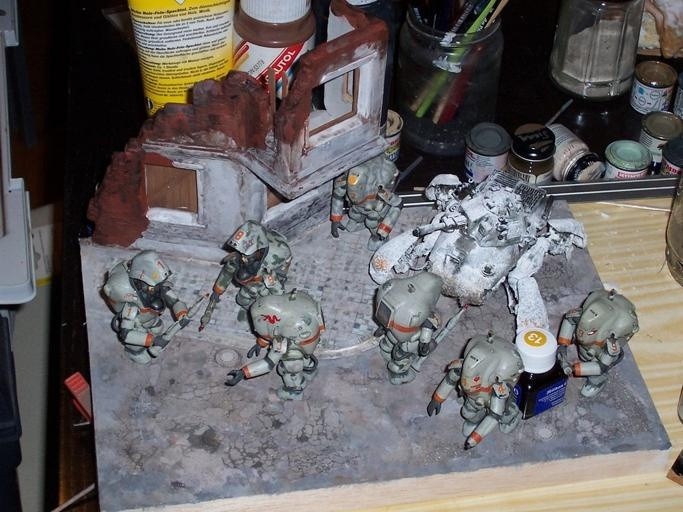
[545,98,574,128]
[410,0,510,124]
[398,156,424,180]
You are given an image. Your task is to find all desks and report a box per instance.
[341,197,682,511]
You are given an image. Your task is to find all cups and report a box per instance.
[393,9,504,157]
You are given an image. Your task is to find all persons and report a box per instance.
[100,248,209,366]
[371,270,469,382]
[426,328,527,451]
[197,218,293,333]
[223,278,326,404]
[555,287,640,398]
[328,155,406,252]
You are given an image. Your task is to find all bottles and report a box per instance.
[550,1,650,97]
[507,327,569,419]
[466,106,649,189]
